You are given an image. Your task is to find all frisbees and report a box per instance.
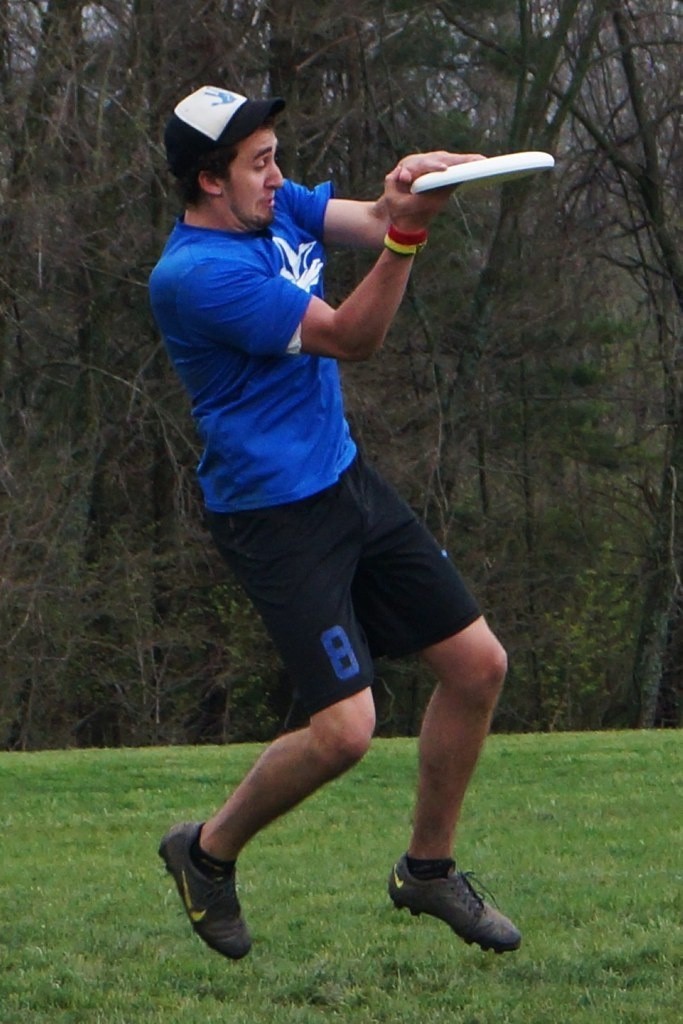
[409,151,556,196]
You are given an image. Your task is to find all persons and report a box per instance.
[151,79,531,958]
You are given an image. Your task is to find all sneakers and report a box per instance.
[158,824,251,958]
[388,855,523,953]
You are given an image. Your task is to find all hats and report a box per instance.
[164,86,285,176]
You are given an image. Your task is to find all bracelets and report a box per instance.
[385,222,430,258]
